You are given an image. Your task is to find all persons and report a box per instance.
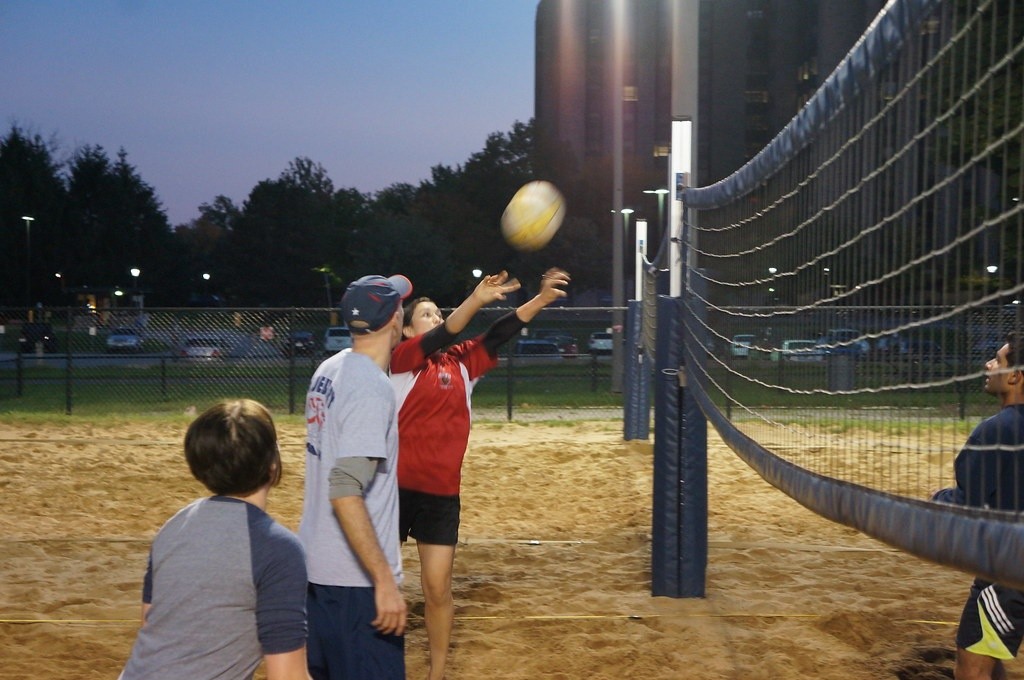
[299,274,413,680]
[117,398,311,680]
[387,269,571,680]
[927,330,1023,680]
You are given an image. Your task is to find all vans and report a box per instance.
[732,334,758,359]
[513,339,563,365]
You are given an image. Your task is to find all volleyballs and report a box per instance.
[499,178,570,255]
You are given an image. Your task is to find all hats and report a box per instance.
[340,274,413,335]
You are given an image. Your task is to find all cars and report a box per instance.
[770,339,824,362]
[106,328,142,351]
[282,332,317,357]
[896,341,965,379]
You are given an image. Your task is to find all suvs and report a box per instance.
[588,332,612,355]
[818,330,869,356]
[180,339,231,368]
[18,322,59,352]
[323,328,353,356]
